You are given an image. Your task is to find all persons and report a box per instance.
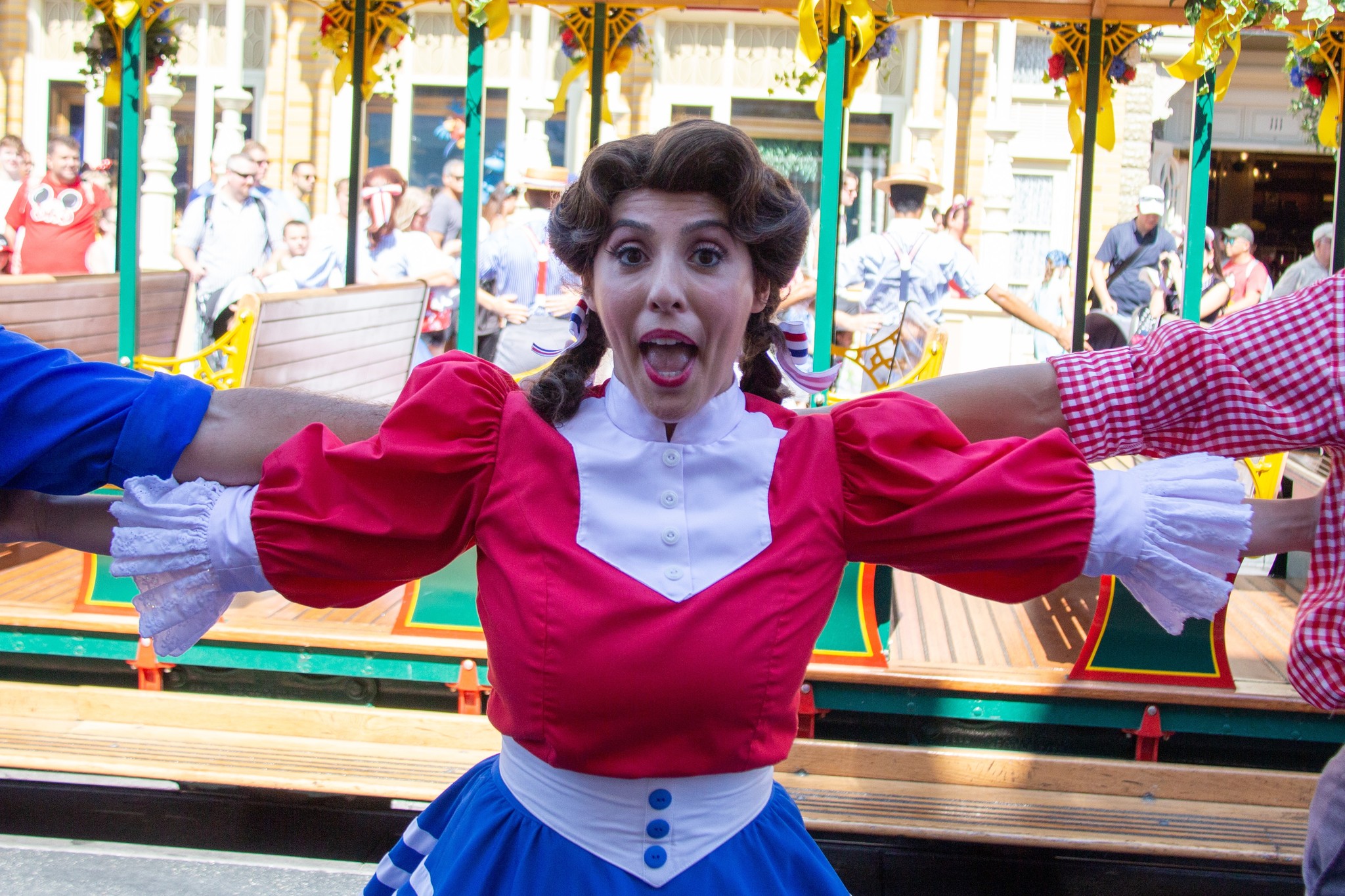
[0,136,1336,393]
[0,116,1323,896]
[2,327,402,495]
[790,271,1345,895]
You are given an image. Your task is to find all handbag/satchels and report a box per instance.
[1087,286,1101,313]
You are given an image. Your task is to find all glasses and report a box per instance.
[848,188,859,194]
[305,175,317,180]
[255,161,270,165]
[455,175,464,180]
[1224,237,1234,246]
[230,170,256,178]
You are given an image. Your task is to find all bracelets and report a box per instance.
[1220,307,1227,317]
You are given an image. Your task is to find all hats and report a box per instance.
[518,166,572,192]
[1312,222,1332,242]
[1138,184,1165,217]
[875,161,943,196]
[1222,222,1254,244]
[1181,223,1215,241]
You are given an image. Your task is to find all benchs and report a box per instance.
[0,266,1332,690]
